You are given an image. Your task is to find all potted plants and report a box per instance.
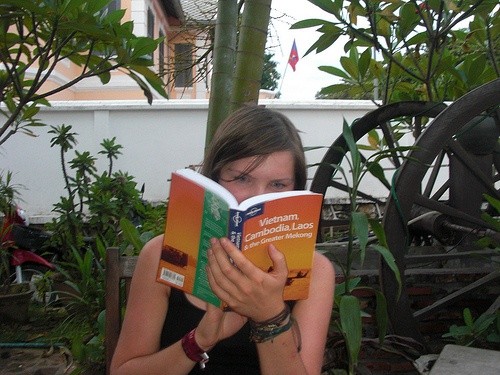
[313,119,431,375]
[47,124,162,375]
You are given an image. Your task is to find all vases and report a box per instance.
[0,283,36,323]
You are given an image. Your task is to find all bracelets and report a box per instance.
[181,327,214,369]
[247,305,293,343]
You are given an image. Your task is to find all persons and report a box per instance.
[108,104,336,375]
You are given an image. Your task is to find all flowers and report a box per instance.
[0,173,56,288]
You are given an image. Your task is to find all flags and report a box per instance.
[288,38,300,73]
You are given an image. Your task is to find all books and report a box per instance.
[155,169,324,313]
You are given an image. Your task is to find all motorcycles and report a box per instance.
[1,211,96,329]
